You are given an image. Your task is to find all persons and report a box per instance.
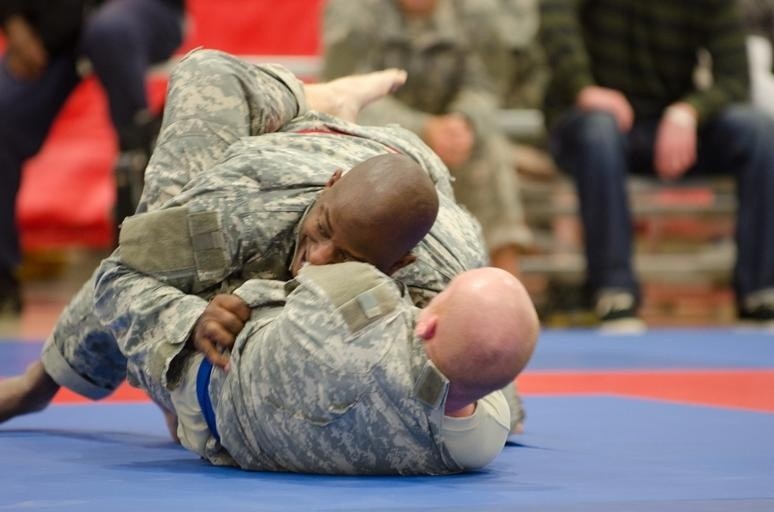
[92,43,542,478]
[0,111,528,448]
[0,0,186,317]
[537,0,772,323]
[493,0,563,234]
[315,0,551,309]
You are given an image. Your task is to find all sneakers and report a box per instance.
[737,287,769,291]
[590,287,647,336]
